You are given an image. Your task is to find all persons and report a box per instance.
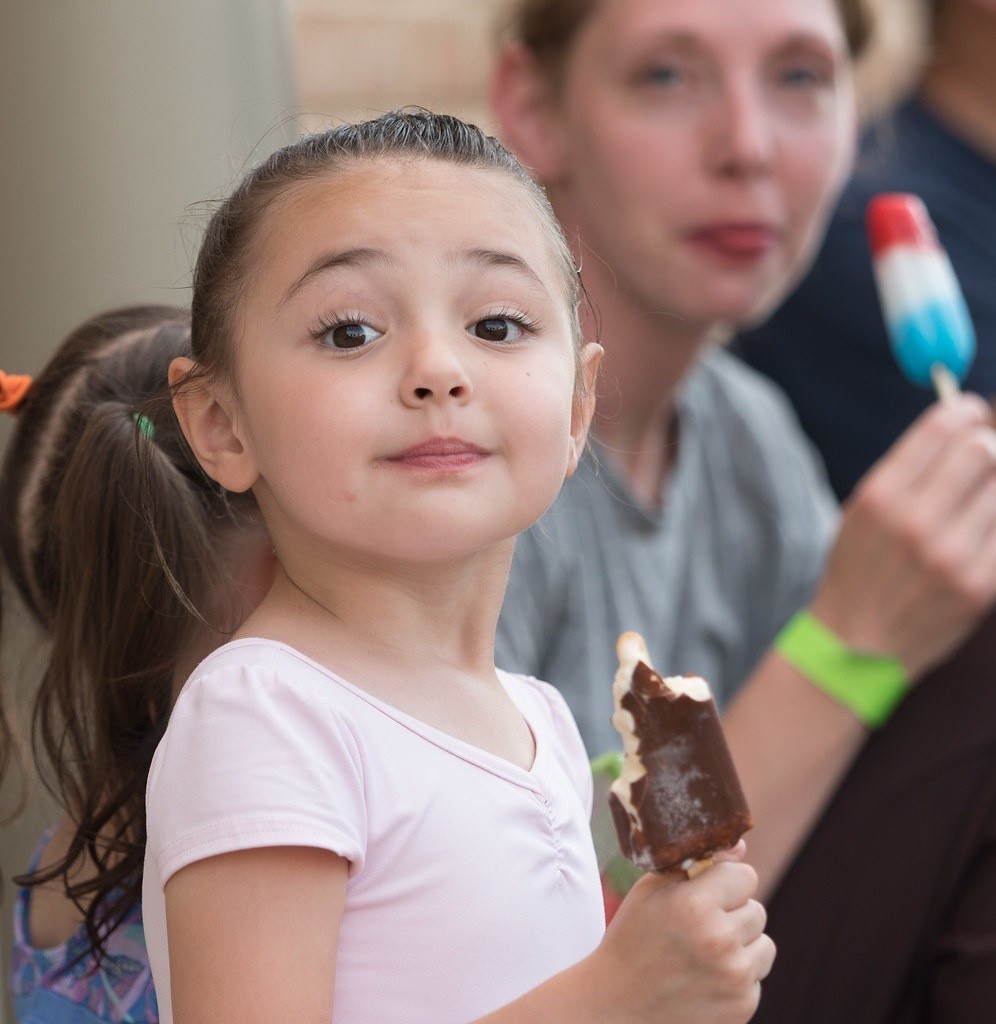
[140,105,777,1024]
[736,0,996,1020]
[482,0,996,920]
[0,307,279,1023]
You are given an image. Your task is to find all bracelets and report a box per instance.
[773,610,912,733]
